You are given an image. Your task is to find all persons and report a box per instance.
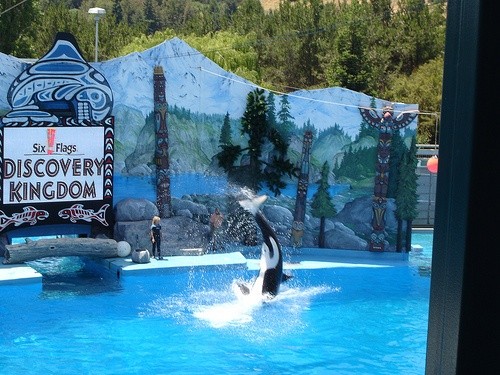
[151,216,163,260]
[211,208,225,253]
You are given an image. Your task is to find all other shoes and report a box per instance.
[159,257,163,260]
[152,256,155,259]
